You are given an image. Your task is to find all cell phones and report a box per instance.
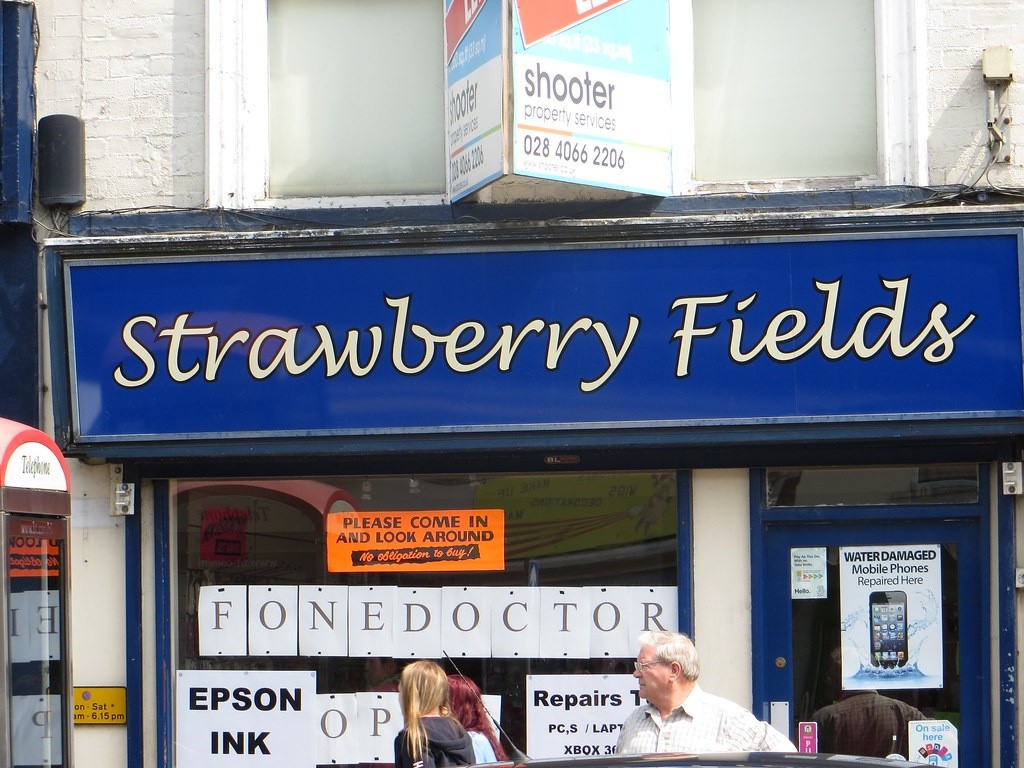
[869,591,908,669]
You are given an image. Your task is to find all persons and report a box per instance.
[446,674,508,765]
[811,646,936,761]
[395,661,476,768]
[613,630,799,755]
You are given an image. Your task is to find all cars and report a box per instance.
[446,749,966,768]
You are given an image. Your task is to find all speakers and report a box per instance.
[37,114,86,209]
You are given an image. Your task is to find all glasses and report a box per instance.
[634,661,665,672]
